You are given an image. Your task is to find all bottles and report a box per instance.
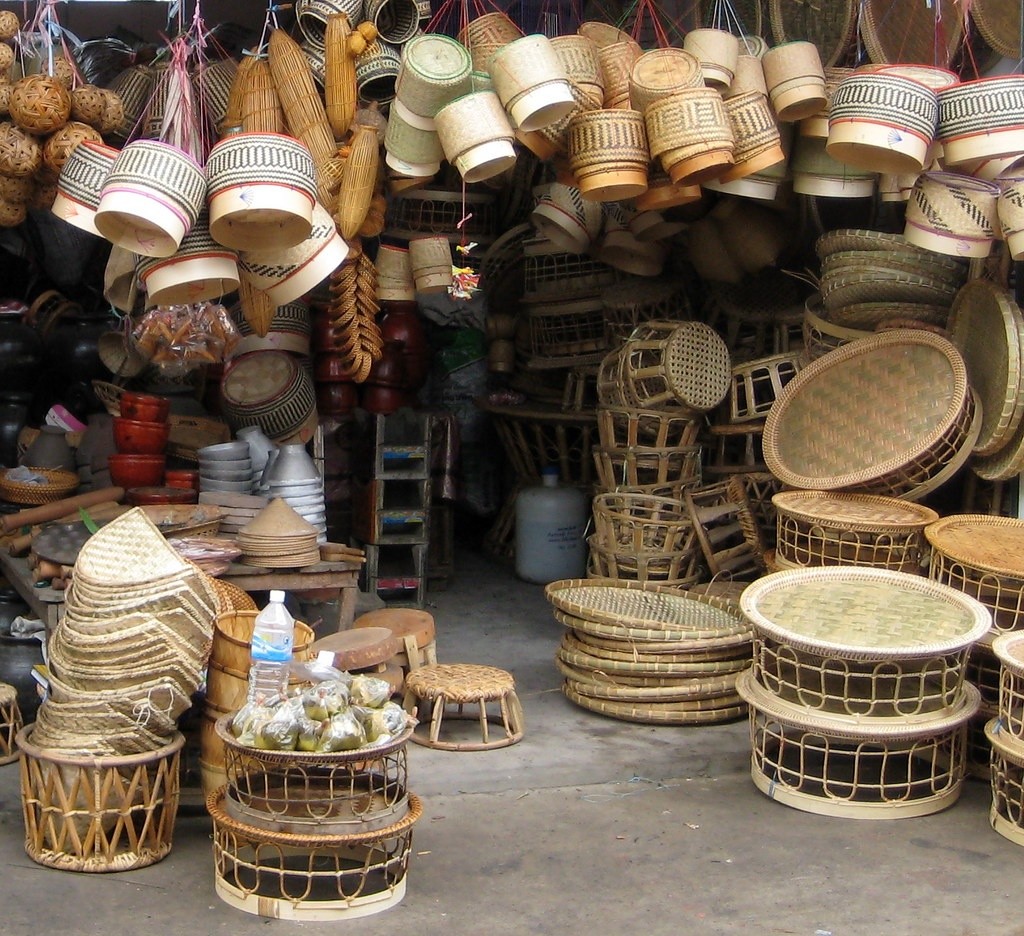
[514,467,586,585]
[248,590,294,711]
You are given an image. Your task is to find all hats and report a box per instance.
[235,495,320,568]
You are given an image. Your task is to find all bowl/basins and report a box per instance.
[236,426,277,494]
[259,444,327,543]
[107,454,166,491]
[315,300,357,415]
[120,392,172,423]
[111,417,172,455]
[360,301,427,413]
[196,442,253,495]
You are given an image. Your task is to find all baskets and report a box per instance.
[27,506,217,856]
[0,467,80,504]
